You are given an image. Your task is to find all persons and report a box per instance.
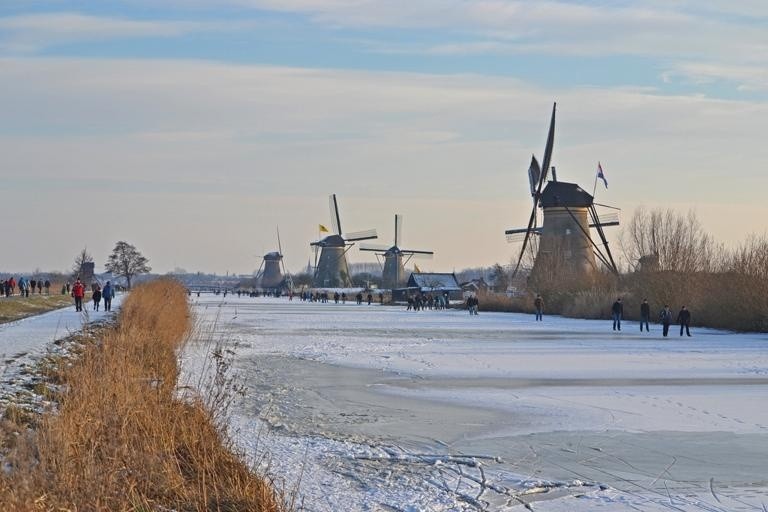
[102,281,114,312]
[534,294,544,321]
[407,293,449,312]
[213,287,384,306]
[612,299,623,330]
[72,277,84,312]
[0,277,70,298]
[467,295,474,315]
[92,286,101,312]
[640,299,649,332]
[473,296,478,315]
[677,305,691,337]
[660,304,672,337]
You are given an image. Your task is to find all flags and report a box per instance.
[414,264,420,273]
[597,162,608,189]
[319,224,328,232]
